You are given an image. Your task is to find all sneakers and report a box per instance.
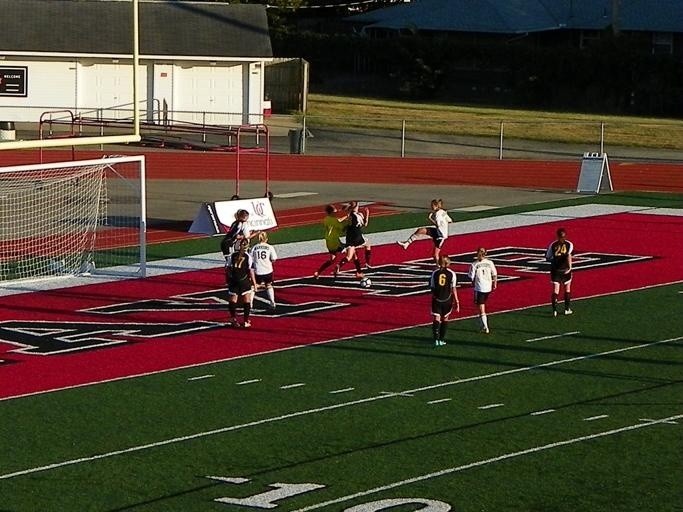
[479,327,490,334]
[243,320,251,327]
[270,302,277,308]
[564,308,572,315]
[553,310,557,317]
[232,321,240,328]
[396,241,407,251]
[313,263,373,281]
[438,340,446,346]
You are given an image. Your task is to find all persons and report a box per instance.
[230,212,250,254]
[228,238,257,328]
[334,201,372,278]
[249,231,278,313]
[468,248,497,333]
[429,255,460,346]
[397,197,453,268]
[314,205,350,280]
[219,209,260,271]
[545,229,573,317]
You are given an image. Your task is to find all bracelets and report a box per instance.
[346,214,349,218]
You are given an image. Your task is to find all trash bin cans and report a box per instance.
[288,128,308,154]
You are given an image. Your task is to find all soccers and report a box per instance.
[361,278,371,288]
